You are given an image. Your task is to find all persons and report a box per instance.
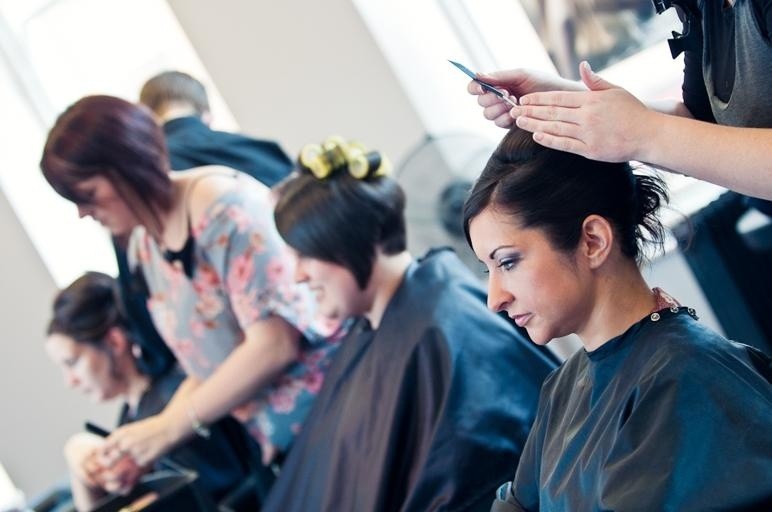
[259,134,566,512]
[465,0,772,200]
[43,271,268,512]
[463,118,772,512]
[106,69,295,379]
[37,93,355,512]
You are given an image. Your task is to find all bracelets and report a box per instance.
[183,401,212,440]
